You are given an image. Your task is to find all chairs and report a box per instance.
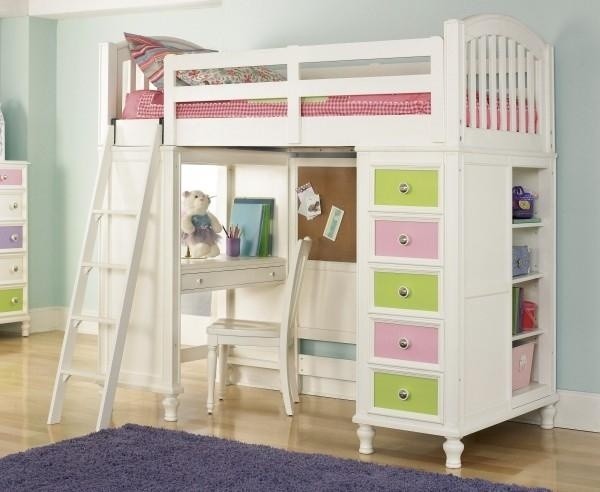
[204,237,314,417]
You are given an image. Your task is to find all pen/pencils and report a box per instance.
[223,224,244,238]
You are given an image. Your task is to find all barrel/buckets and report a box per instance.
[520,301,537,331]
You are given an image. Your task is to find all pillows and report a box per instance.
[123,32,288,93]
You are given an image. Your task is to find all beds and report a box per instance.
[98,13,556,157]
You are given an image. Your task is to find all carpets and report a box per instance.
[0,422,552,492]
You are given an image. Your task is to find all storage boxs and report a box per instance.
[510,339,541,394]
[511,246,530,278]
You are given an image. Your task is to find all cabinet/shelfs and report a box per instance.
[370,317,444,371]
[371,215,442,267]
[0,160,32,340]
[368,366,441,424]
[371,166,446,213]
[368,264,442,317]
[464,165,557,422]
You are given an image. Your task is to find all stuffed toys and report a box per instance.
[181,191,225,260]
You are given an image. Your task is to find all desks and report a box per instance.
[98,254,300,424]
